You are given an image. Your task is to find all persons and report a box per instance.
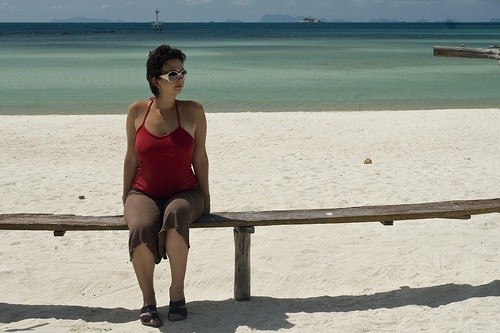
[123,44,211,328]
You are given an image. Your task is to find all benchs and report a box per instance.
[0,198,500,300]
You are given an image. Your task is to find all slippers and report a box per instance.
[168,286,187,321]
[140,305,162,327]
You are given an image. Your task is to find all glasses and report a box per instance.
[159,69,187,81]
